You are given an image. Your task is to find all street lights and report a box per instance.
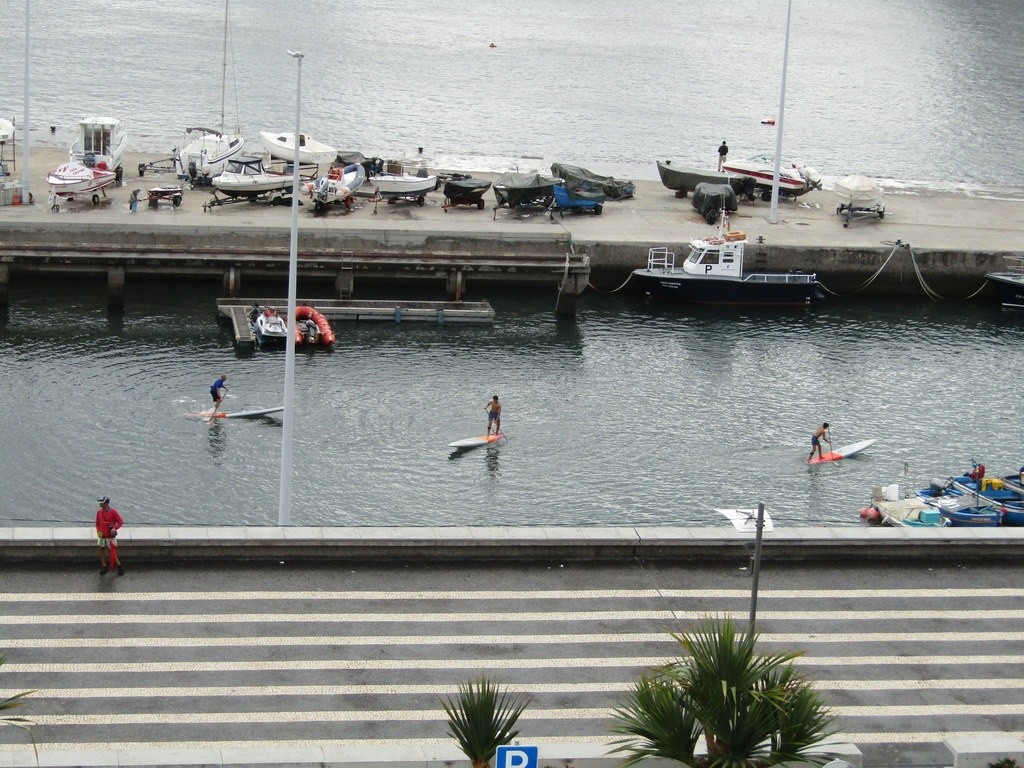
[278,48,303,531]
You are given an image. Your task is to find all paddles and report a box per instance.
[207,388,228,422]
[827,426,834,462]
[486,408,508,442]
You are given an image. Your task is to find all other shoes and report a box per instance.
[100,566,108,575]
[118,563,123,575]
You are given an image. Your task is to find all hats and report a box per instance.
[97,496,107,503]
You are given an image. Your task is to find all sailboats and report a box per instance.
[176,1,245,181]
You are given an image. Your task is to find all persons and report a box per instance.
[807,423,830,462]
[717,141,728,173]
[210,375,228,409]
[484,395,501,436]
[95,496,124,576]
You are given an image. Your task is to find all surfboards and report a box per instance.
[449,433,506,447]
[188,407,284,418]
[804,438,876,464]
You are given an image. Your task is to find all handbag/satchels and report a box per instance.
[110,529,117,537]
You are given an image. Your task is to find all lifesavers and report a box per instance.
[704,236,724,245]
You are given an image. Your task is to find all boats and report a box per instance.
[722,153,822,195]
[68,116,129,169]
[443,175,492,201]
[369,174,438,198]
[696,180,732,209]
[552,162,630,199]
[554,184,605,209]
[210,151,294,194]
[259,128,337,165]
[984,256,1024,309]
[253,309,286,349]
[0,118,14,144]
[45,161,116,197]
[301,160,351,189]
[835,173,885,209]
[496,172,566,202]
[631,211,825,306]
[859,461,1024,527]
[657,160,757,197]
[294,305,335,348]
